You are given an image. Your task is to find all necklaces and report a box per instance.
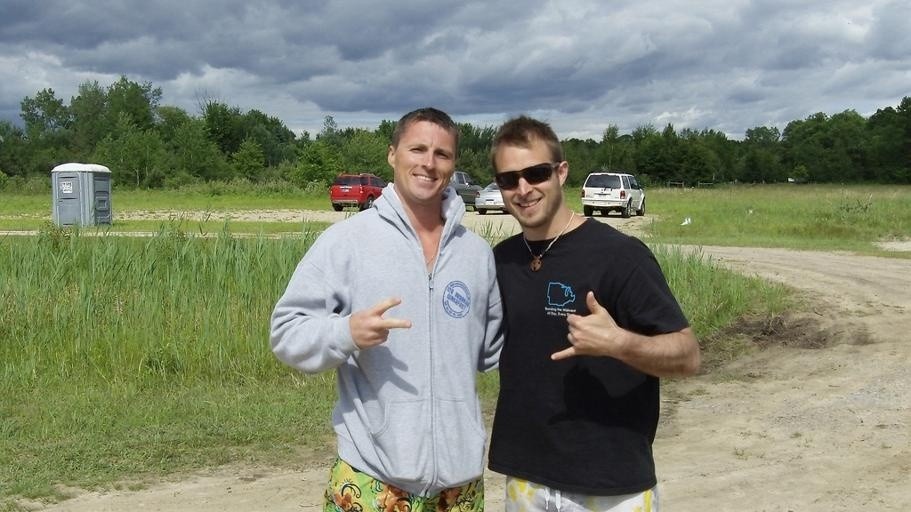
[425,237,441,265]
[521,209,577,271]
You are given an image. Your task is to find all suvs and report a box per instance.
[329,171,388,212]
[580,172,646,218]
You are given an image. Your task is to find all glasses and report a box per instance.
[495,163,556,189]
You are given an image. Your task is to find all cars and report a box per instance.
[447,171,510,215]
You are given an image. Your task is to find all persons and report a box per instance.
[490,113,700,512]
[270,108,507,512]
